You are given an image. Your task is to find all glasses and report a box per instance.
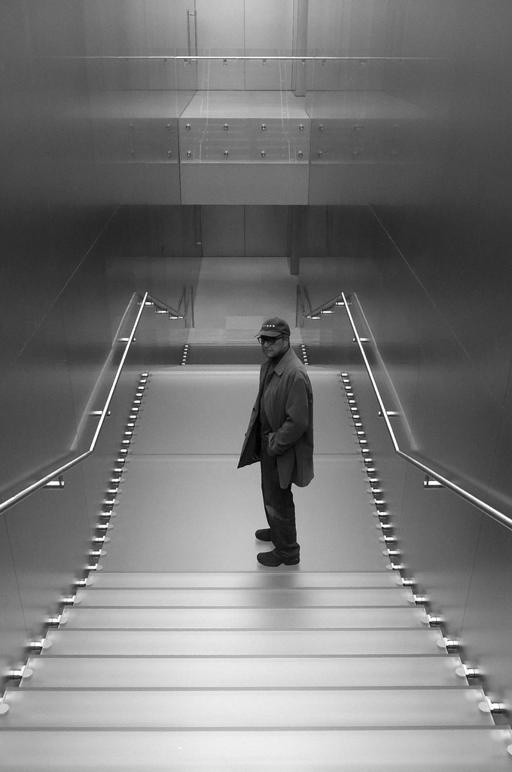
[257,337,283,344]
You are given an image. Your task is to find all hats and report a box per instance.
[255,318,290,337]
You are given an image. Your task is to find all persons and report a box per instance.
[234,316,316,568]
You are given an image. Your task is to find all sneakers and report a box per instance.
[255,529,272,541]
[257,548,299,566]
